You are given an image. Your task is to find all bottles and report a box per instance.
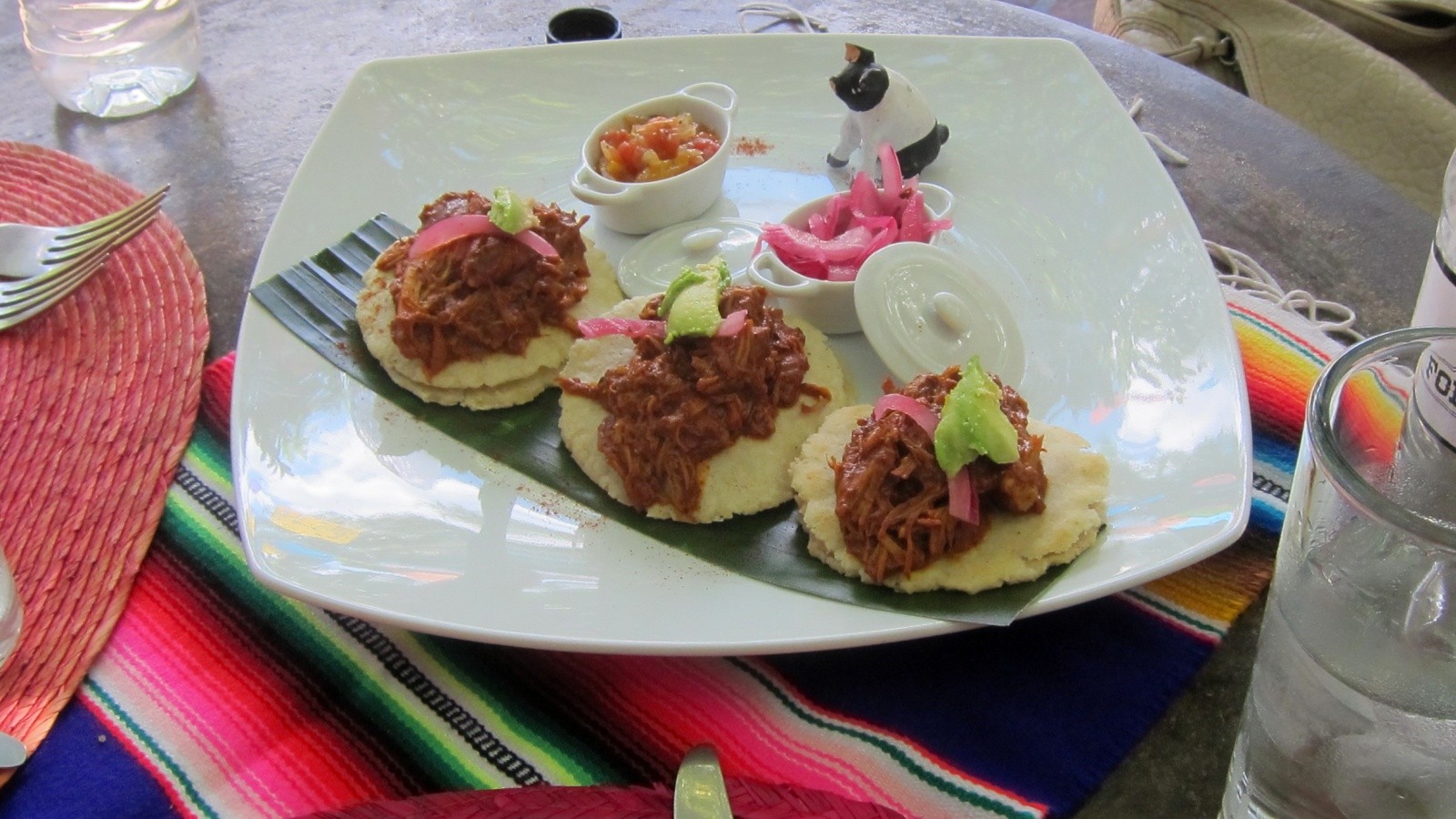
[20,0,203,116]
[1392,146,1455,487]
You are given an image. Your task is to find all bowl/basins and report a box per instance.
[747,179,955,336]
[568,81,736,234]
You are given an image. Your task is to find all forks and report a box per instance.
[0,232,123,331]
[0,181,171,276]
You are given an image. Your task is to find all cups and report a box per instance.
[1220,321,1456,819]
[547,9,622,45]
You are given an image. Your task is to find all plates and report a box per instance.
[233,34,1261,653]
